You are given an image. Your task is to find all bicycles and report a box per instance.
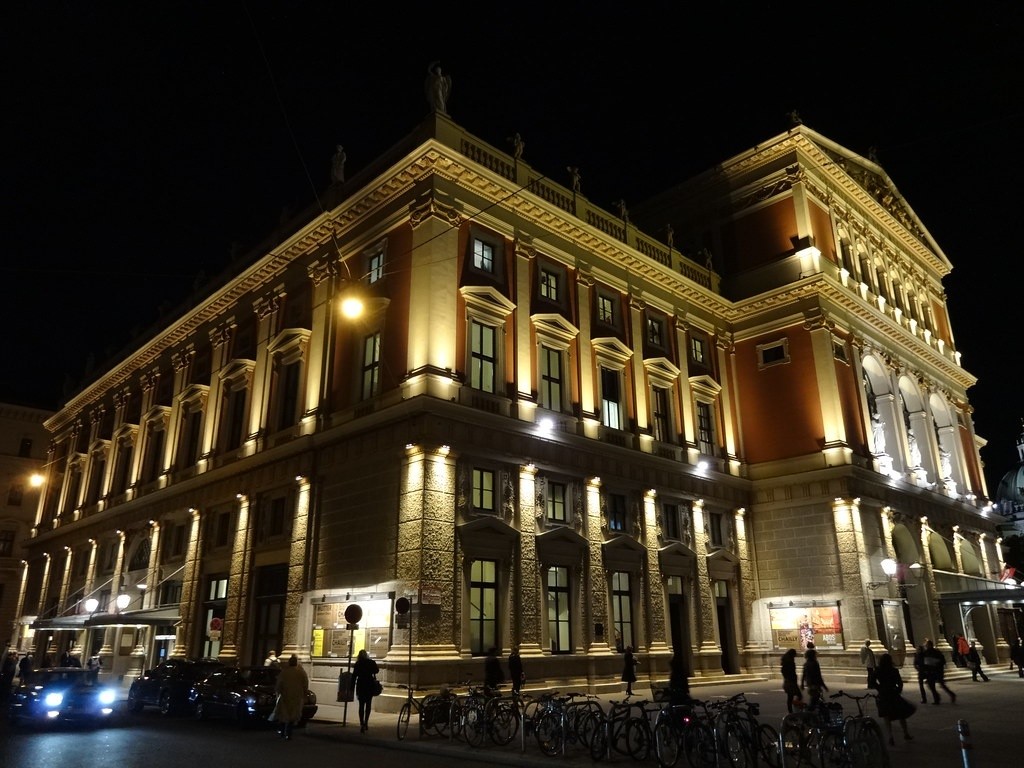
[396,676,894,768]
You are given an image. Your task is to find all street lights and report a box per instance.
[833,589,846,651]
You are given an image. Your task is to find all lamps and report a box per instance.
[864,559,897,589]
[299,595,304,604]
[345,592,351,600]
[895,562,925,589]
[322,594,326,602]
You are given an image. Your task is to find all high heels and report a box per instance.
[625,690,633,695]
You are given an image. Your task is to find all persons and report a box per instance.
[17,652,34,687]
[483,647,505,704]
[60,647,70,667]
[669,639,694,694]
[267,656,309,741]
[2,650,19,691]
[800,641,830,706]
[350,650,379,731]
[871,653,917,745]
[949,630,991,683]
[621,646,641,696]
[780,649,803,713]
[860,639,879,690]
[1003,623,1024,680]
[913,638,956,705]
[508,645,525,697]
[87,649,103,678]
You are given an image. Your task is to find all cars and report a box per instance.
[5,653,100,726]
[190,653,319,729]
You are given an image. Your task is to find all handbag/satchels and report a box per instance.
[373,680,383,697]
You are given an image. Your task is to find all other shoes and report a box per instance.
[360,724,369,731]
[951,693,955,704]
[276,729,290,742]
[984,678,991,683]
[889,739,895,747]
[921,700,926,704]
[904,735,912,741]
[973,678,980,683]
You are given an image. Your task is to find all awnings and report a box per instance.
[83,604,179,628]
[29,608,150,631]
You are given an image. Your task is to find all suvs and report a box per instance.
[127,654,226,717]
